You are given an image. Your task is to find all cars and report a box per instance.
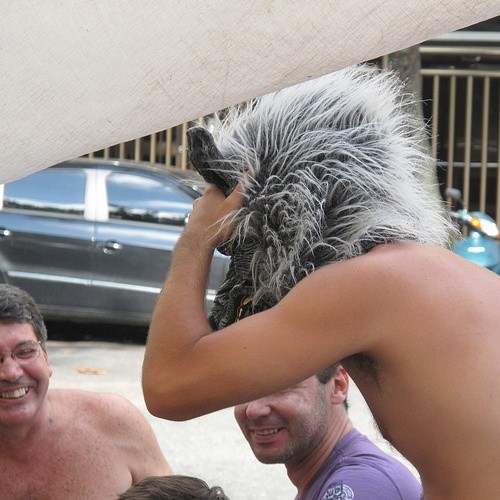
[0,156,243,336]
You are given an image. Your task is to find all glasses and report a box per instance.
[0,340,42,366]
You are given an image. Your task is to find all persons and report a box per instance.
[233,360,423,499]
[139,65,499,500]
[0,283,172,500]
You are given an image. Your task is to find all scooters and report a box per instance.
[441,190,500,279]
